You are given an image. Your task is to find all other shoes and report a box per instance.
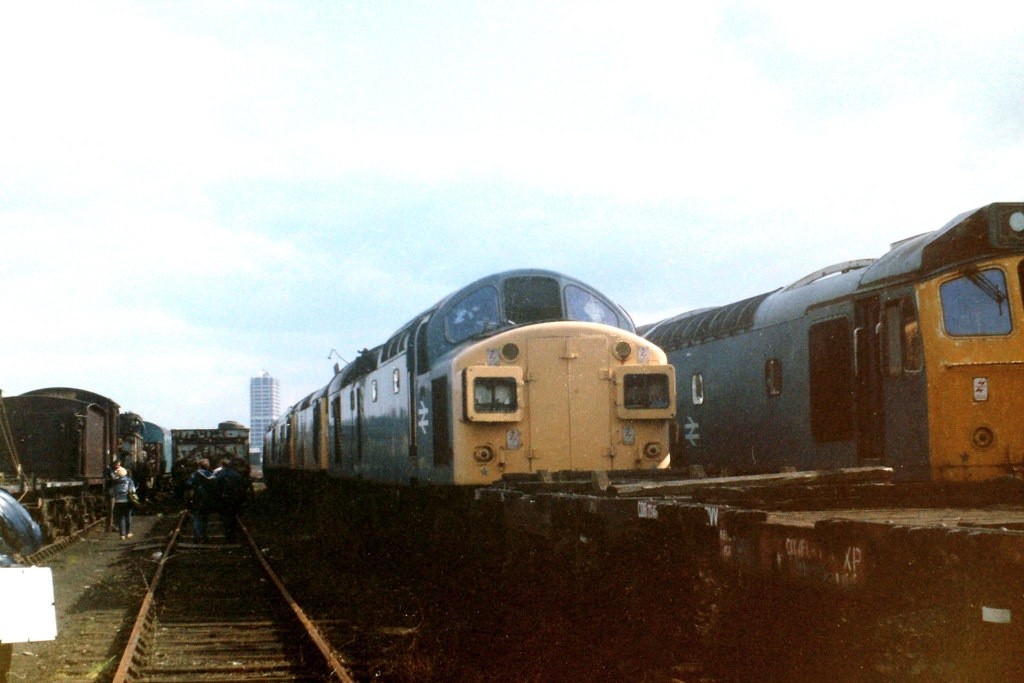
[120,536,125,540]
[204,538,211,544]
[194,536,200,544]
[126,533,134,538]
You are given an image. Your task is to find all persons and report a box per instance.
[189,458,250,541]
[103,455,155,541]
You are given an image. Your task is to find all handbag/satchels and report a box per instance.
[128,480,139,505]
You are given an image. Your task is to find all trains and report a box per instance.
[636,200,1024,484]
[260,266,679,502]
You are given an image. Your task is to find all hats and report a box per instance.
[115,467,127,476]
